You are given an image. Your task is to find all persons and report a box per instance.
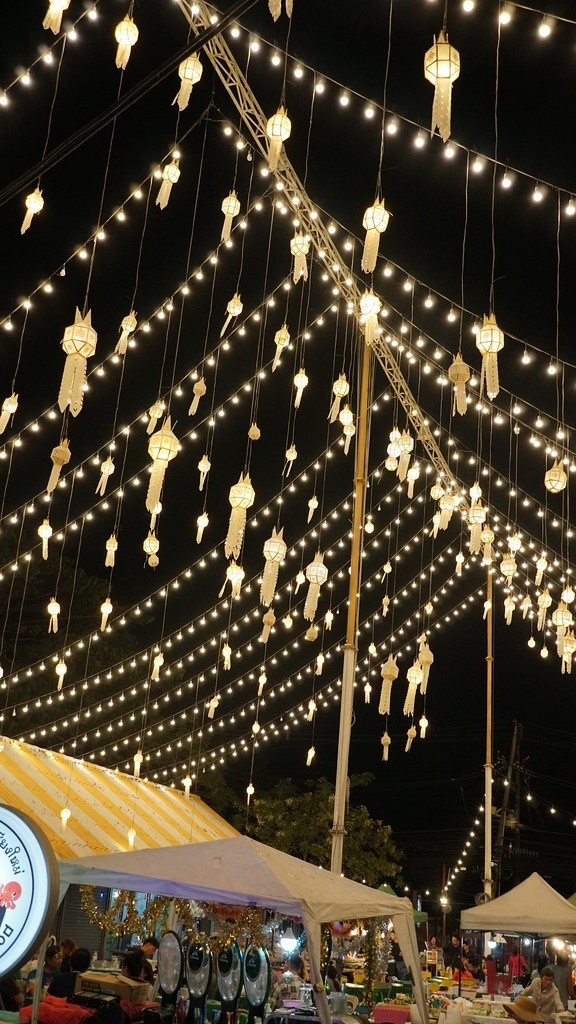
[509,946,528,983]
[530,951,576,1011]
[390,932,410,981]
[427,935,485,983]
[513,967,565,1014]
[122,938,159,986]
[503,996,545,1024]
[121,909,138,950]
[326,965,342,1004]
[344,935,357,956]
[0,936,91,1012]
[269,953,307,1024]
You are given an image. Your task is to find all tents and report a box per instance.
[30,836,430,1024]
[378,885,428,973]
[458,872,576,997]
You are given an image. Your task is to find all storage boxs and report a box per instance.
[75,971,150,1006]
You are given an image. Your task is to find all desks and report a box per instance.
[236,960,576,1024]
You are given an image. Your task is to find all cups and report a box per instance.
[300,984,315,1007]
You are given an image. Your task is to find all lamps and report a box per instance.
[0,0,576,897]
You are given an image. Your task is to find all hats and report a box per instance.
[503,996,546,1022]
